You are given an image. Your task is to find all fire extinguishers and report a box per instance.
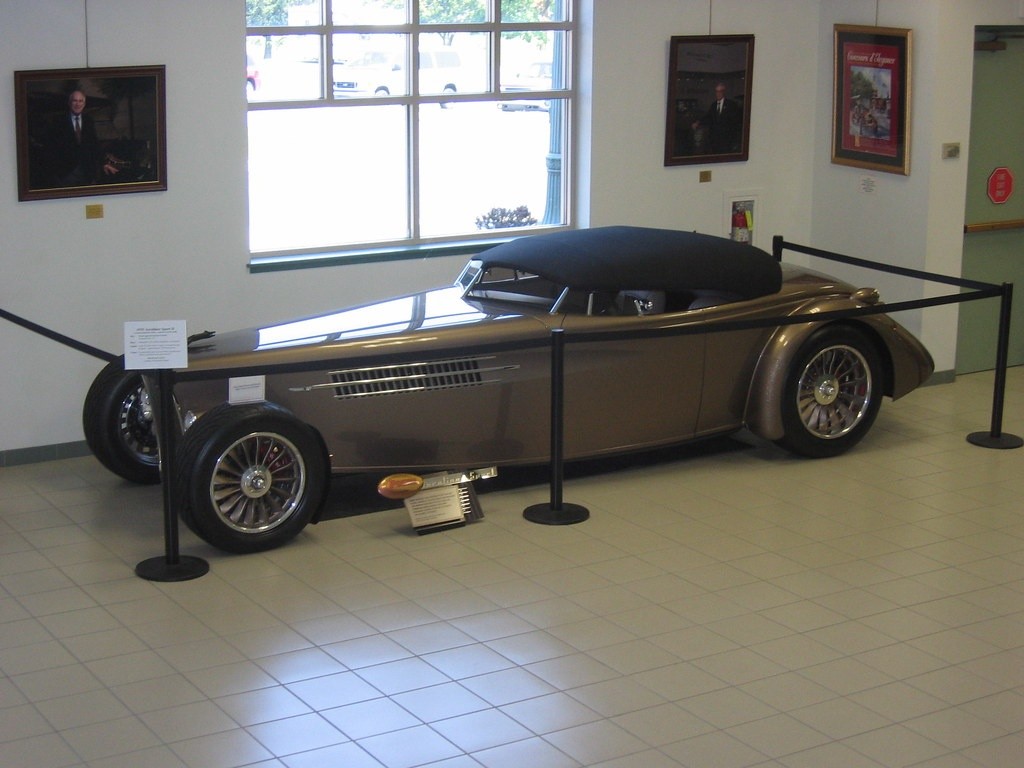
[732,202,752,245]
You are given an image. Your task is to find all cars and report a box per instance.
[247,46,554,115]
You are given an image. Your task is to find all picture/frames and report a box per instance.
[14,64,168,202]
[831,24,911,177]
[664,34,755,166]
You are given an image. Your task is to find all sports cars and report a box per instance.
[83,226,936,555]
